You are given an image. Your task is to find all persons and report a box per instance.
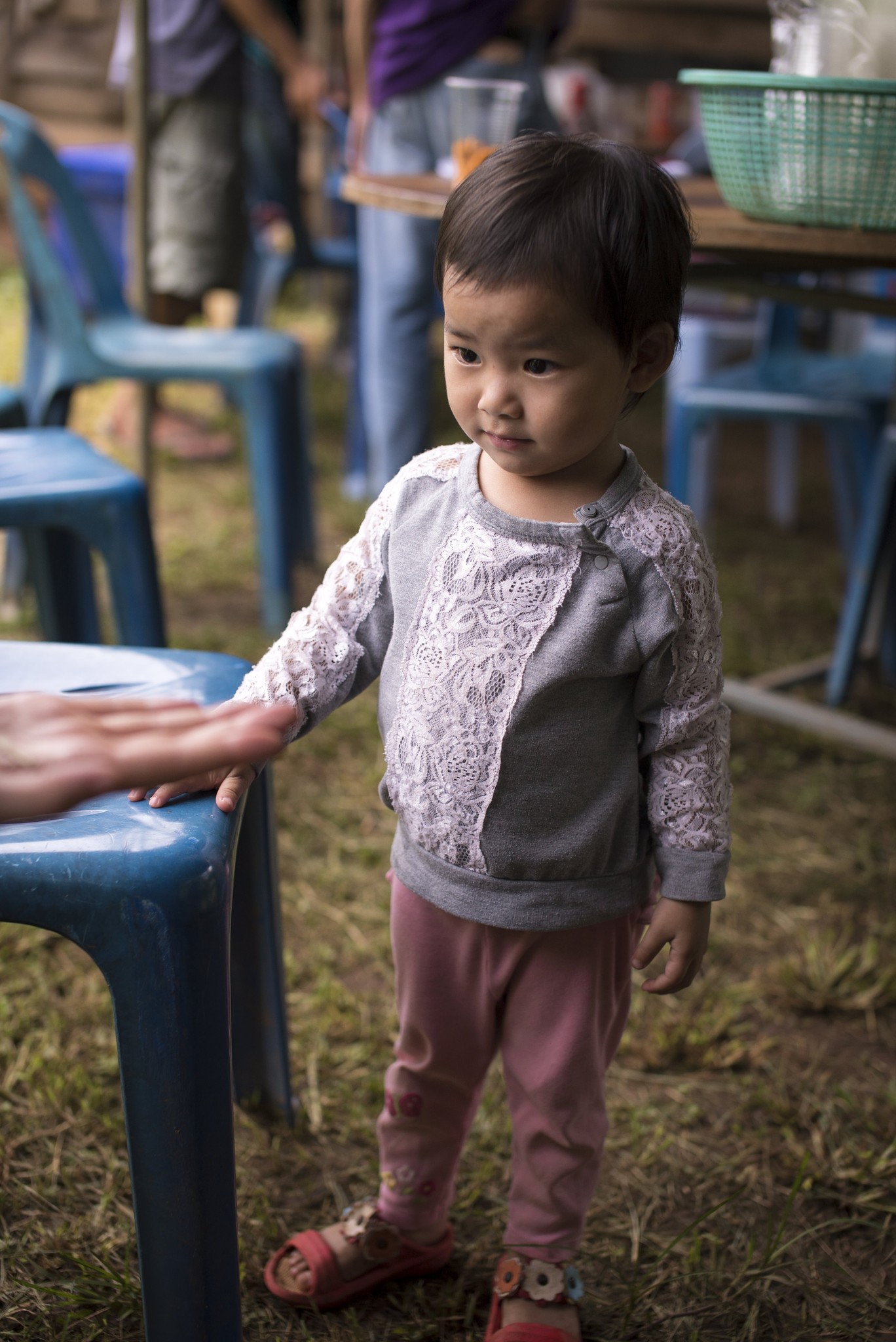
[91,0,570,496]
[126,128,730,1342]
[0,691,294,819]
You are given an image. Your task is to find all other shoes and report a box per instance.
[102,379,238,461]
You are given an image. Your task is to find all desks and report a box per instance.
[336,173,896,762]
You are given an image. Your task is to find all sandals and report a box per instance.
[486,1253,581,1342]
[264,1203,453,1306]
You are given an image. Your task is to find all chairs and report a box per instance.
[0,642,294,1342]
[0,103,312,631]
[670,268,896,703]
[0,422,170,643]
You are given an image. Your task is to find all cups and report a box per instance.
[445,75,528,190]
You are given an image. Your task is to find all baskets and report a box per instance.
[679,69,896,230]
